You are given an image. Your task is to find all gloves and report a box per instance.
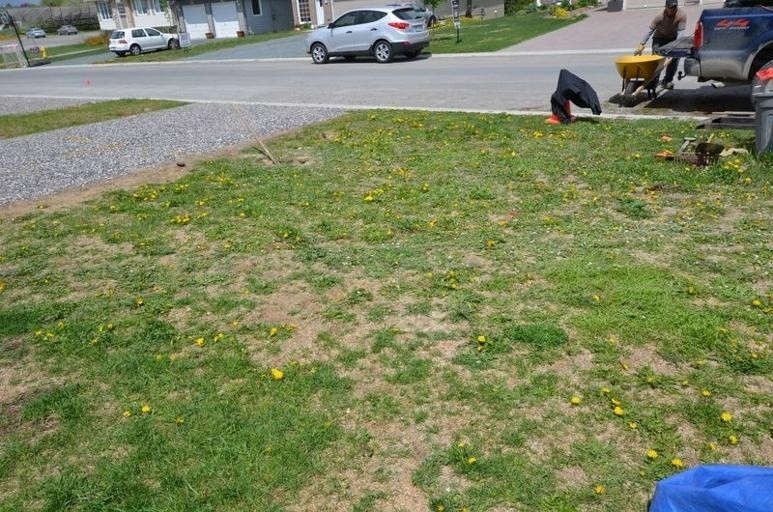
[632,44,645,56]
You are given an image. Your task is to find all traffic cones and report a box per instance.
[545,99,577,126]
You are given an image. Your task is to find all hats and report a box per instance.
[666,0,678,9]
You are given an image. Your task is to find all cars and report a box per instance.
[305,3,439,64]
[56,25,78,35]
[108,26,179,57]
[26,27,46,38]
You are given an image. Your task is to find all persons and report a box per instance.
[633,0,687,89]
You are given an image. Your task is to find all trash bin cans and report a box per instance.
[750,94,772,154]
[39,48,45,58]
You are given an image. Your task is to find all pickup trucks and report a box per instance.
[655,0,773,112]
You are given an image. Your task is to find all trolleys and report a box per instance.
[614,46,673,107]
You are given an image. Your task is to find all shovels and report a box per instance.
[695,132,724,155]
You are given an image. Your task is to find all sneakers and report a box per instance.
[659,81,675,89]
